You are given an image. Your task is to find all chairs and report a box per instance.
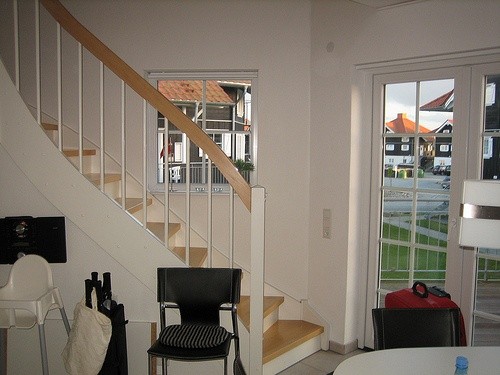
[147,267,247,375]
[372,309,462,350]
[0,252,70,375]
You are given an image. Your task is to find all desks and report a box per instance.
[333,346,500,375]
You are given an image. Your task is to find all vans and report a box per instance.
[392,163,414,173]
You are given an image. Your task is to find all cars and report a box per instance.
[444,165,451,175]
[169,163,239,184]
[432,166,446,175]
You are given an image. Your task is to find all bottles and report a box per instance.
[454,356,468,375]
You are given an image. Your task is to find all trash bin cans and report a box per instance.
[169,165,182,184]
